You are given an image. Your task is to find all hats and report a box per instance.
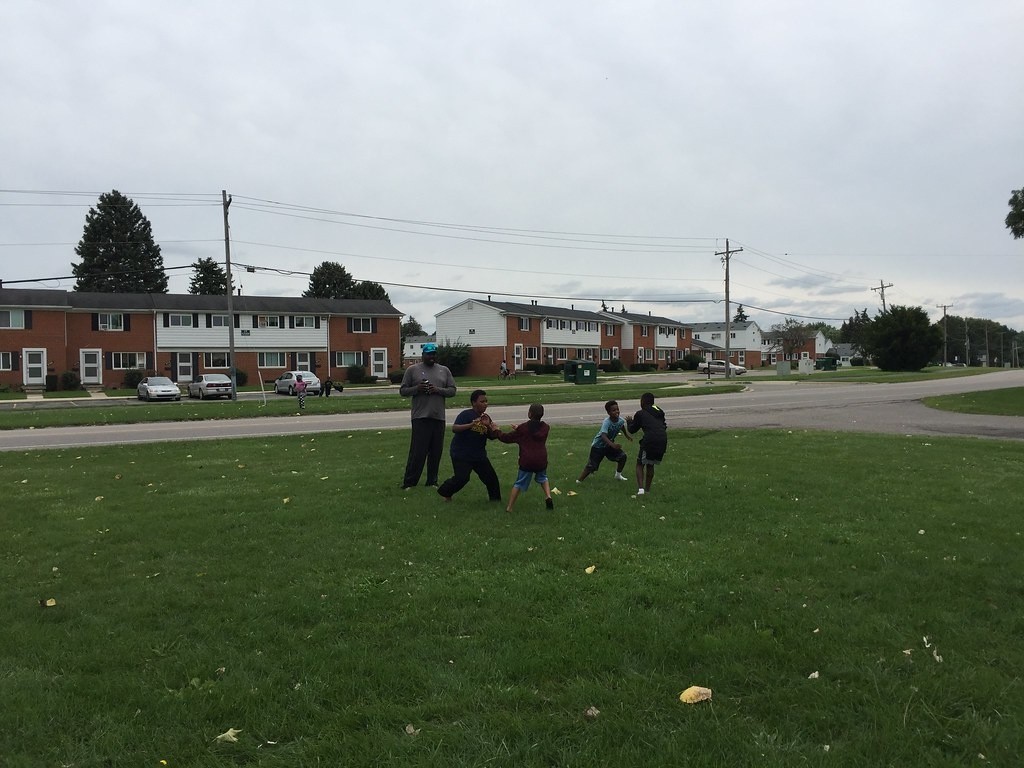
[423,344,437,353]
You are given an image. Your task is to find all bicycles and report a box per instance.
[497,368,516,380]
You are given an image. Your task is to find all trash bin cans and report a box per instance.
[568,374,575,383]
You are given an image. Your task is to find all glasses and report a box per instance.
[424,346,436,351]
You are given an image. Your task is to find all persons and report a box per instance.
[323,375,335,398]
[437,390,501,503]
[399,343,457,491]
[576,400,633,484]
[489,403,554,512]
[293,375,307,409]
[500,360,509,378]
[624,393,667,495]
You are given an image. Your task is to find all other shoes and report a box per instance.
[546,498,553,509]
[615,475,627,481]
[638,489,644,495]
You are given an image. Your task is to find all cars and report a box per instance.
[697,360,747,375]
[136,376,181,402]
[187,373,232,399]
[273,370,321,395]
